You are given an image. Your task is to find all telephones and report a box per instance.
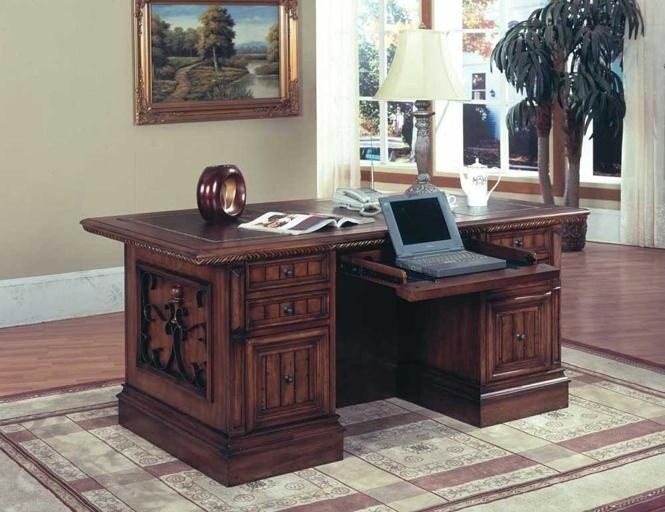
[334,187,387,208]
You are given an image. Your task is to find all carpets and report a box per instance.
[0,337,665,512]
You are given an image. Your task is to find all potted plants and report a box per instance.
[494,0,647,251]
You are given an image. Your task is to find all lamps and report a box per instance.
[371,27,475,215]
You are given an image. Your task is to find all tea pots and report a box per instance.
[458,157,502,209]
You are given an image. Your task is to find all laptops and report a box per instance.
[376,190,507,280]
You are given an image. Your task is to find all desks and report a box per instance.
[80,188,590,489]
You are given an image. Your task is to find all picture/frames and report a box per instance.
[132,0,302,131]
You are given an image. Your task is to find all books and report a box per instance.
[236,206,376,240]
